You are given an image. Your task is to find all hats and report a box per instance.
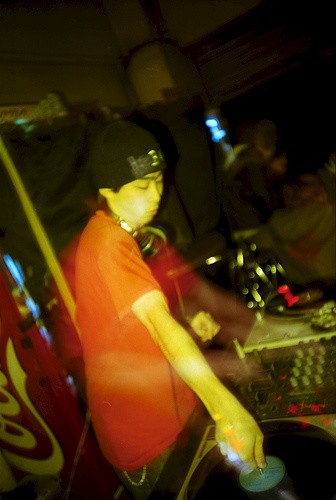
[90,120,167,188]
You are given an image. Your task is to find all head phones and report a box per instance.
[104,207,167,258]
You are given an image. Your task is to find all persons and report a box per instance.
[53,196,280,387]
[218,115,336,284]
[74,120,268,500]
